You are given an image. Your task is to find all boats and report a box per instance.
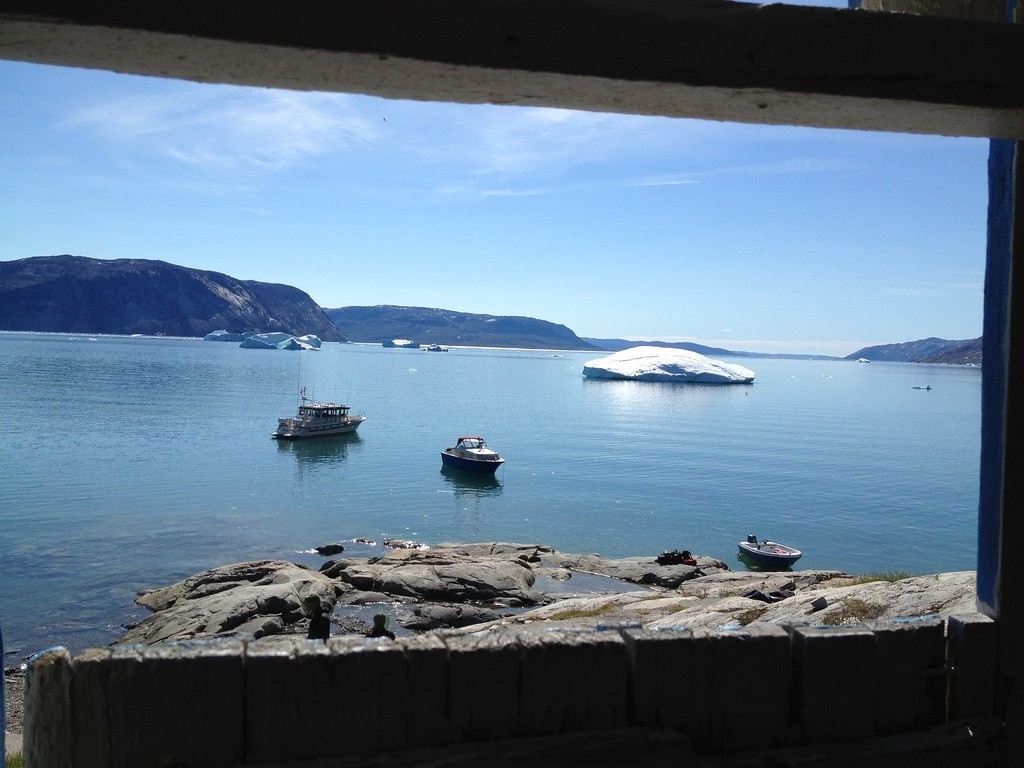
[271,388,367,441]
[440,437,505,475]
[737,534,803,567]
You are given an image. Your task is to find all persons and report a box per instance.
[305,594,331,641]
[365,613,395,640]
